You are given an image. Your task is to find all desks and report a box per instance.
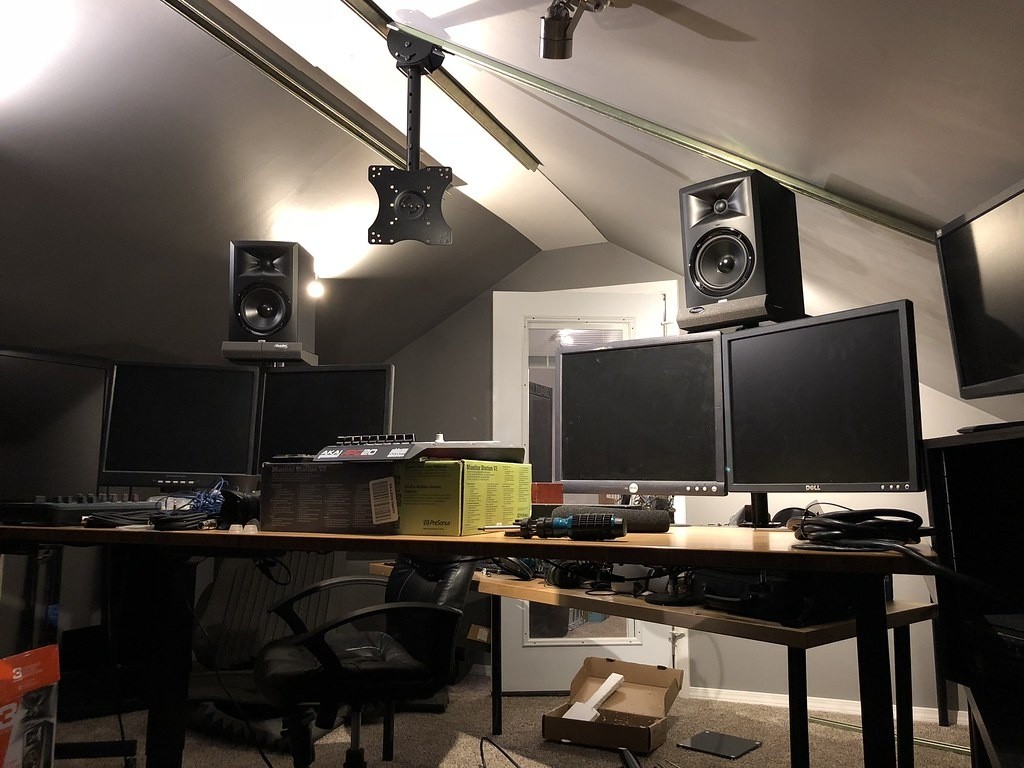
[0,526,941,768]
[366,561,939,768]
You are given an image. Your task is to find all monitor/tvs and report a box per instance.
[0,350,394,518]
[934,178,1024,399]
[726,299,924,494]
[553,331,726,495]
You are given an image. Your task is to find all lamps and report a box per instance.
[538,1,608,60]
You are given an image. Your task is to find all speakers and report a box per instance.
[222,241,318,368]
[675,169,805,331]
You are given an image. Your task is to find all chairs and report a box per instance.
[250,552,481,768]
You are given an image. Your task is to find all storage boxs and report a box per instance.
[258,462,399,535]
[399,459,533,536]
[0,644,61,768]
[540,657,685,753]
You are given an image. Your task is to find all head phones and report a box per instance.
[494,555,603,589]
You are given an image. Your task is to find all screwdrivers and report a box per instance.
[475,512,628,543]
